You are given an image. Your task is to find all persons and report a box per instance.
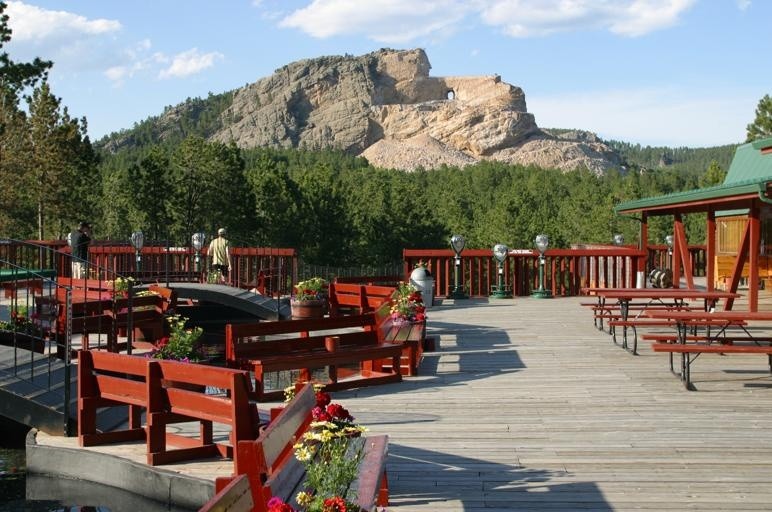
[70,220,92,278]
[207,227,232,280]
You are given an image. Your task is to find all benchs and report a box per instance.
[1,274,428,512]
[713,255,772,293]
[581,287,771,390]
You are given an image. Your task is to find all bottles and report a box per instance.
[218,227,227,236]
[79,221,89,230]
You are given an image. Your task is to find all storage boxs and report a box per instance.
[713,255,772,293]
[581,287,771,390]
